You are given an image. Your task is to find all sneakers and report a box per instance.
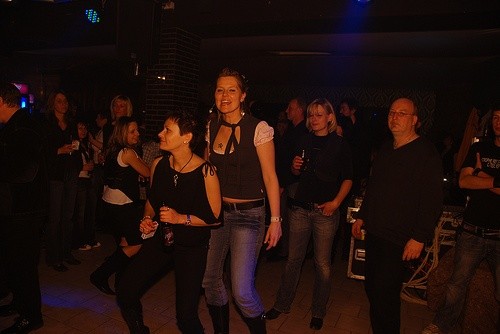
[421,323,447,334]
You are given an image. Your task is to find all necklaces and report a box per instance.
[172,153,193,187]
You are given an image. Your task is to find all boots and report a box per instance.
[204,300,232,334]
[87,255,118,295]
[241,312,267,334]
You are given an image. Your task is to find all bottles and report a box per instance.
[160,202,175,246]
[300,148,308,171]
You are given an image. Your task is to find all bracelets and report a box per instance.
[143,216,151,221]
[472,168,482,177]
[271,216,282,224]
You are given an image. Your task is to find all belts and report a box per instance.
[461,221,500,241]
[220,199,265,214]
[286,195,321,211]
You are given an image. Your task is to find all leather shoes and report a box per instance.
[264,304,290,321]
[0,297,20,316]
[309,312,324,330]
[47,252,83,273]
[0,314,43,334]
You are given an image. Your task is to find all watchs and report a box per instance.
[185,214,192,226]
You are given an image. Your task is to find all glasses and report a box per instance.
[215,86,240,96]
[388,110,415,119]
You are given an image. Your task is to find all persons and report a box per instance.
[89,116,151,296]
[352,98,443,334]
[1,79,363,333]
[421,109,500,334]
[201,68,282,334]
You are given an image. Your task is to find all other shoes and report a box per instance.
[79,239,102,251]
